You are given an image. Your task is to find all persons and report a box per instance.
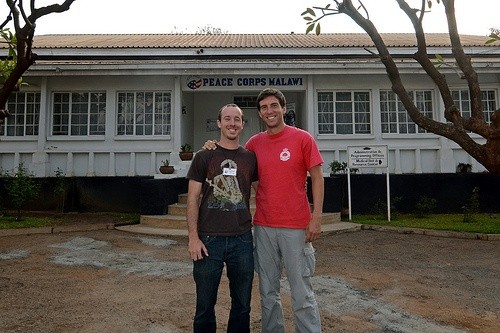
[202,87,325,333]
[186,103,260,332]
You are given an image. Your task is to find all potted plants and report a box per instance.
[342,162,358,174]
[327,160,342,177]
[160,160,174,174]
[179,144,194,161]
